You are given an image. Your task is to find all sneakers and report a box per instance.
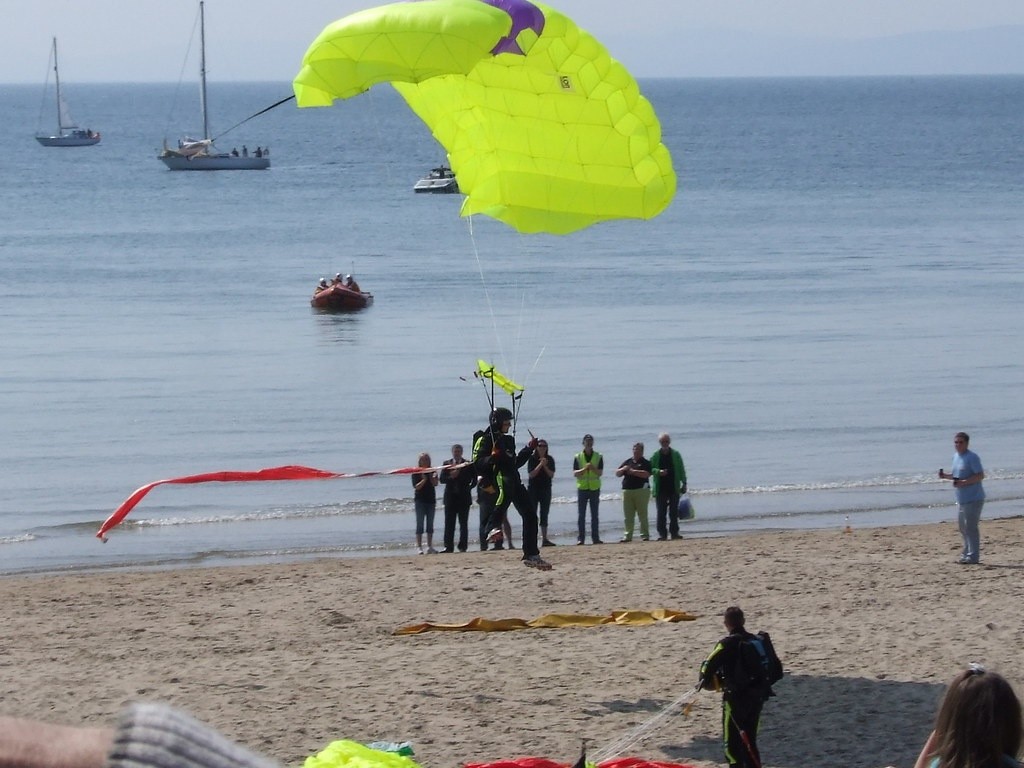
[486,527,503,544]
[522,554,552,572]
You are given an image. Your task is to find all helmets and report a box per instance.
[489,407,514,431]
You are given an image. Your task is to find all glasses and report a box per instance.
[538,445,547,448]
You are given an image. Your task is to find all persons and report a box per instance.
[440,165,446,179]
[573,434,604,546]
[440,445,476,553]
[242,145,247,158]
[412,452,439,555]
[476,478,516,551]
[650,433,687,540]
[939,432,986,564]
[527,439,556,546]
[912,668,1024,768]
[87,128,100,140]
[231,147,239,157]
[616,443,651,542]
[313,273,359,295]
[701,606,783,768]
[252,147,262,158]
[473,408,552,571]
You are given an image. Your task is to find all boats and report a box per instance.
[413,164,459,194]
[310,285,375,312]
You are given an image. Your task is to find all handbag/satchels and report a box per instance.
[677,489,695,520]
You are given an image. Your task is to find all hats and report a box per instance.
[583,434,593,440]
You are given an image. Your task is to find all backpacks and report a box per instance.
[722,631,784,693]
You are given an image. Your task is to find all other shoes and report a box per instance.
[671,532,683,539]
[490,546,504,550]
[427,547,438,554]
[643,537,649,541]
[439,546,454,553]
[593,540,603,544]
[416,547,423,554]
[658,535,667,541]
[966,558,979,564]
[620,540,632,542]
[460,549,466,552]
[509,547,515,549]
[957,557,967,563]
[542,539,555,546]
[577,541,584,545]
[481,549,486,551]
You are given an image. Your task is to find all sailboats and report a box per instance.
[34,37,101,147]
[157,1,271,170]
